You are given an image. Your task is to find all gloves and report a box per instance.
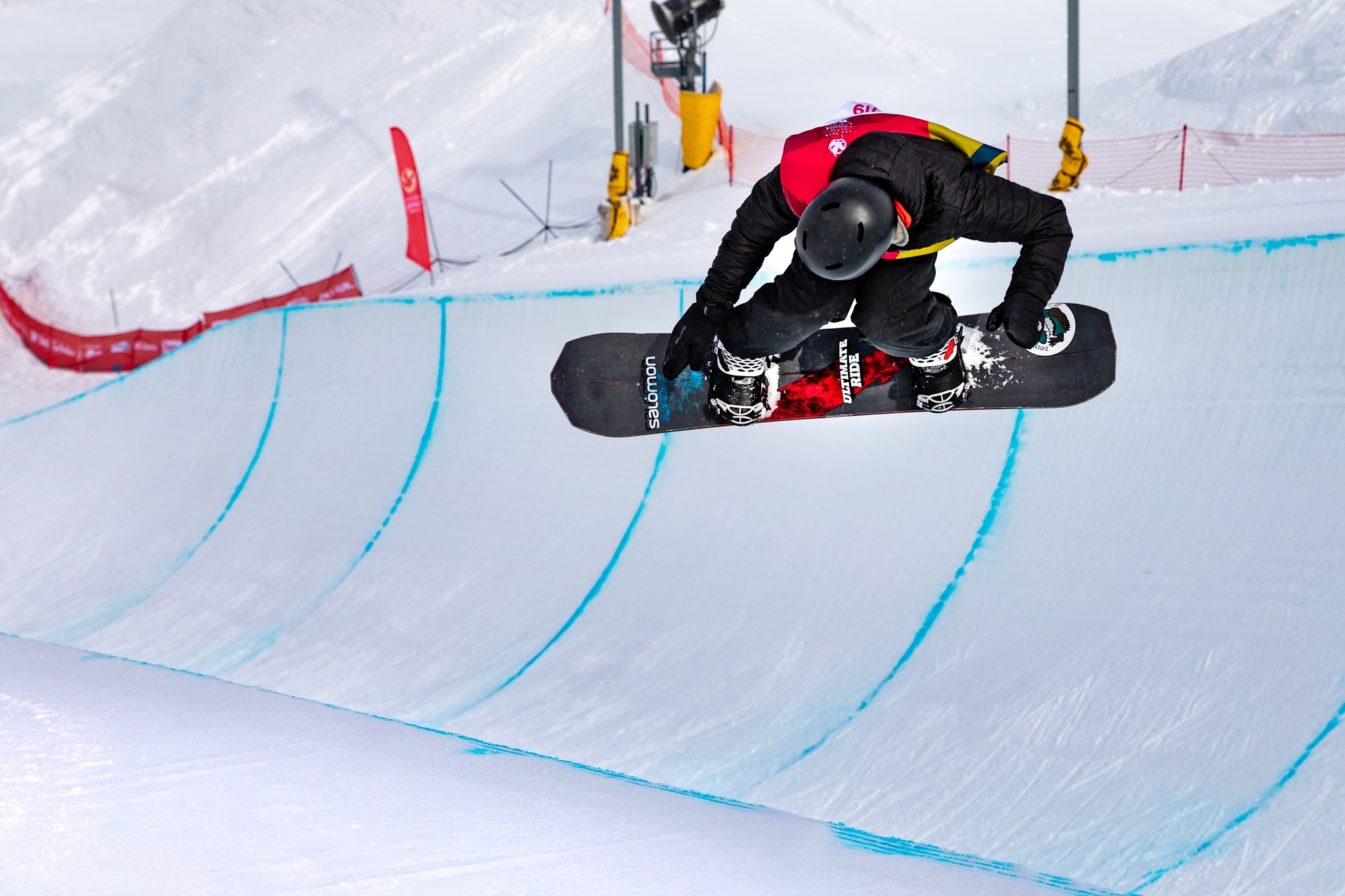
[986,277,1052,349]
[663,293,732,381]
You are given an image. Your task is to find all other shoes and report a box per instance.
[709,334,767,426]
[908,291,967,413]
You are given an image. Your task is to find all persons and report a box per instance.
[662,99,1073,426]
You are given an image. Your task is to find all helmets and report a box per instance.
[795,177,898,281]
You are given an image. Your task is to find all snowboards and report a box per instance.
[550,302,1116,438]
